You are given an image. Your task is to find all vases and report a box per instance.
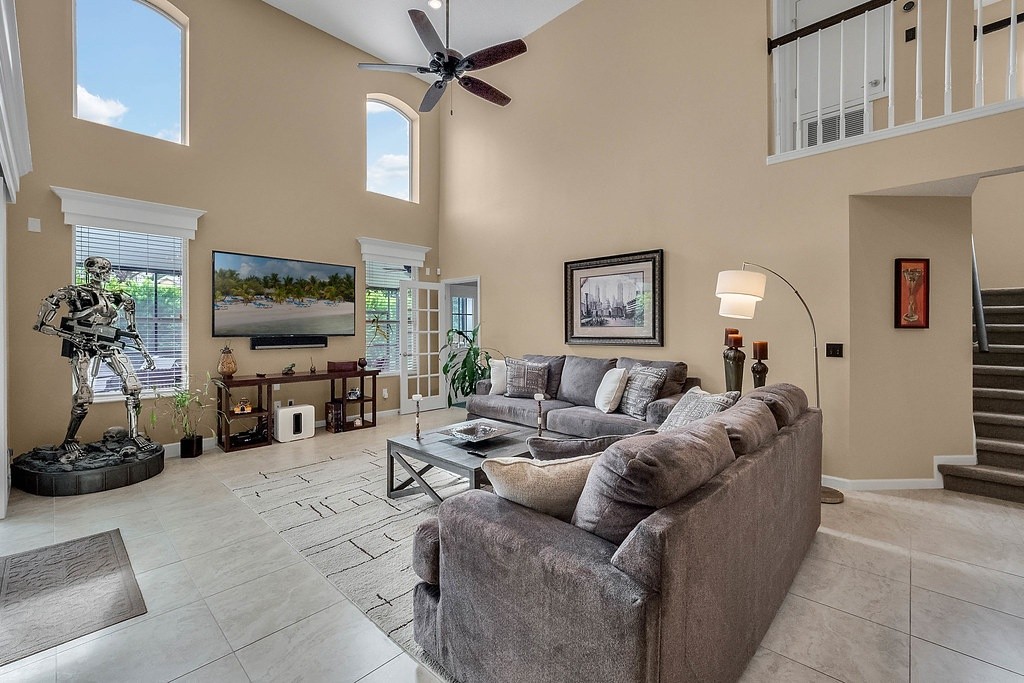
[358,357,368,368]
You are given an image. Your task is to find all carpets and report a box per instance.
[0,528,147,668]
[222,450,495,683]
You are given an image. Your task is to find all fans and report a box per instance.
[356,0,527,115]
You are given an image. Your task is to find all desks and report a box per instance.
[386,416,563,508]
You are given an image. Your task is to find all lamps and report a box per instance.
[715,260,844,503]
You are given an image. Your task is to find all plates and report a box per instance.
[438,422,520,442]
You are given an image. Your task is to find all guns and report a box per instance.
[58,315,141,360]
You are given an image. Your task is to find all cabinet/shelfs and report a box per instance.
[211,367,381,453]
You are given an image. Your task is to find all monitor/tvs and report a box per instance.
[210,250,355,337]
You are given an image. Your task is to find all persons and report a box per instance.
[32,256,155,456]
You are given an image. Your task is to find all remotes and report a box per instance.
[467,450,487,458]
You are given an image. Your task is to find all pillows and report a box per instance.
[557,355,618,407]
[525,430,658,461]
[571,419,738,545]
[619,363,668,420]
[481,451,603,524]
[595,367,628,414]
[523,354,566,399]
[488,359,507,395]
[746,383,808,431]
[658,386,740,432]
[705,397,778,459]
[617,357,687,400]
[503,356,551,399]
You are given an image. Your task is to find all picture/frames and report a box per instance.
[564,248,664,344]
[894,257,930,329]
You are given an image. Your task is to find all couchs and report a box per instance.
[466,377,701,439]
[412,406,823,683]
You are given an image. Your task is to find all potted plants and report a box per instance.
[135,368,233,459]
